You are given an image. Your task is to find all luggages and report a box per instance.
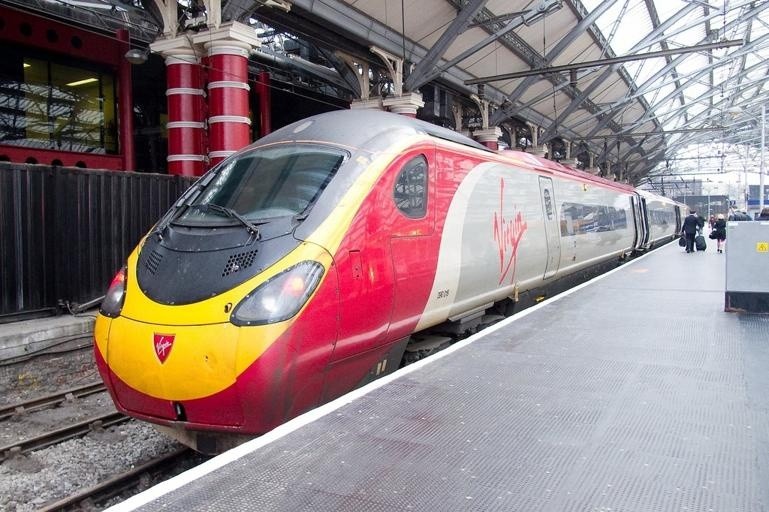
[695,233,706,251]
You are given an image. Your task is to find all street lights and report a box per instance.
[729,103,765,210]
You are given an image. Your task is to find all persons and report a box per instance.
[680,203,769,252]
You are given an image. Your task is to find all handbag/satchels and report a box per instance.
[709,227,718,240]
[678,234,687,247]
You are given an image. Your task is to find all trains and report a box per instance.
[93,110,693,456]
